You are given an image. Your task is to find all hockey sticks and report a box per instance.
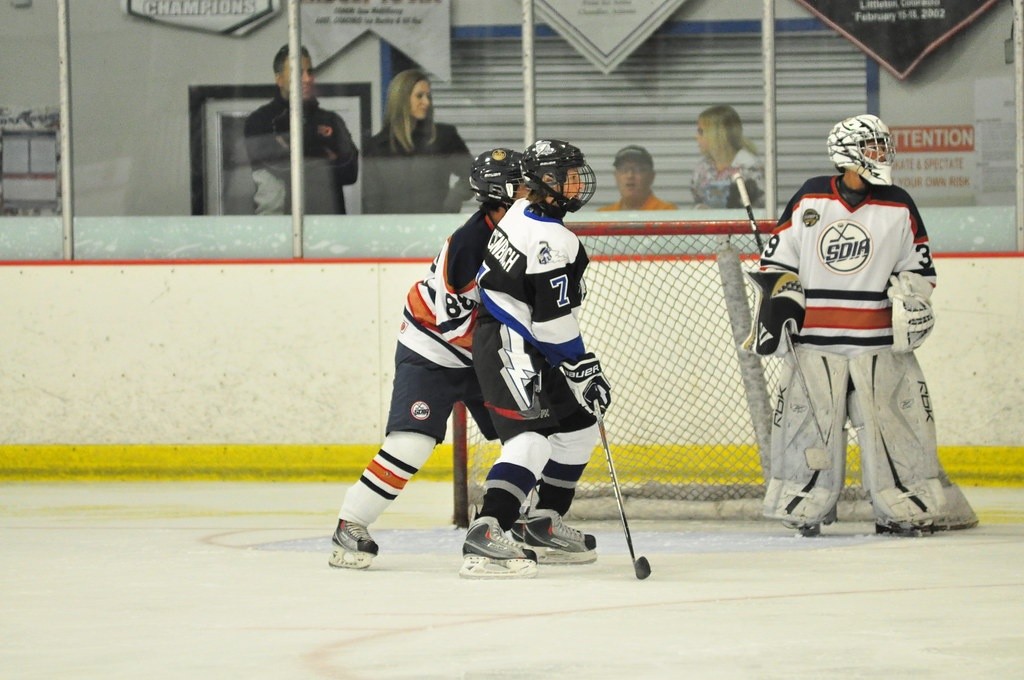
[594,398,651,581]
[733,170,832,471]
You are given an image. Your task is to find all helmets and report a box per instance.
[826,115,896,186]
[520,140,597,212]
[469,148,531,209]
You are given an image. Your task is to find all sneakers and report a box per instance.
[798,502,837,537]
[875,519,934,535]
[328,519,379,569]
[522,509,598,565]
[459,505,537,579]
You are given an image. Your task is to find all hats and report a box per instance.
[613,145,653,166]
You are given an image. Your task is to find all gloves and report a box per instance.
[747,270,805,357]
[558,352,611,418]
[887,271,934,303]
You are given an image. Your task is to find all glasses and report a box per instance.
[616,167,651,173]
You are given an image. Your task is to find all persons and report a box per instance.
[598,146,678,210]
[741,114,978,536]
[459,139,612,577]
[244,42,360,215]
[690,105,766,207]
[327,149,540,570]
[361,68,476,213]
